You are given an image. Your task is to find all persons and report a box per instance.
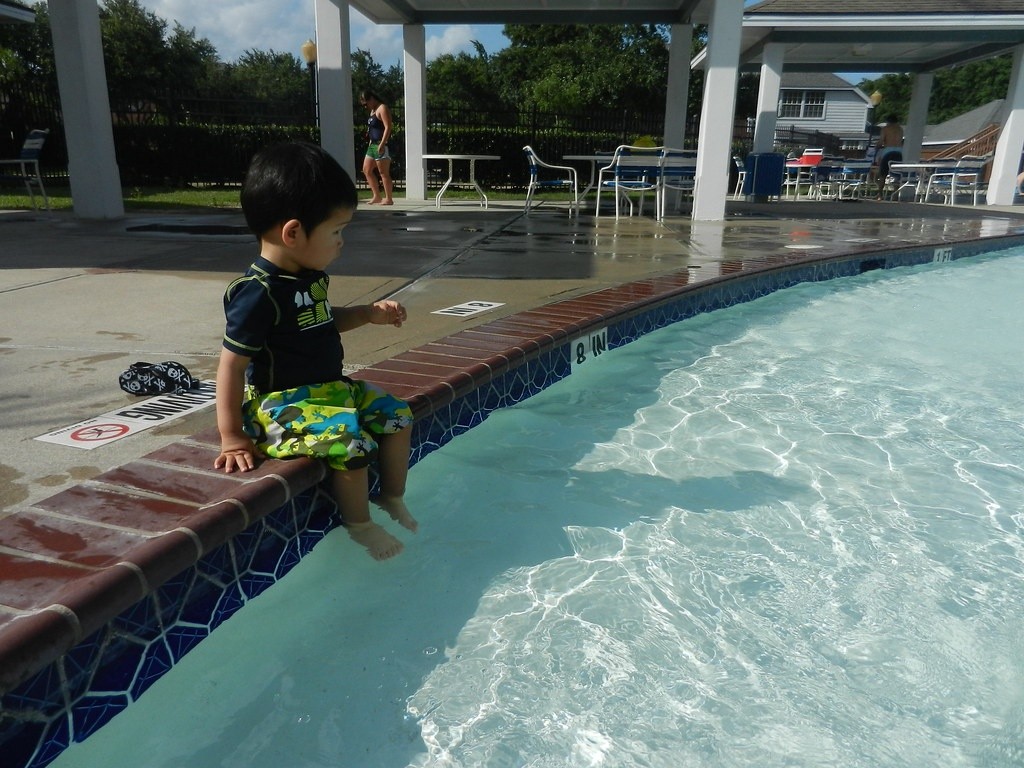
[211,137,422,559]
[359,89,393,205]
[874,114,904,201]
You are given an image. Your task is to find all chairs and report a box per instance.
[785,154,986,207]
[523,145,579,216]
[732,155,747,199]
[656,147,698,217]
[0,128,50,215]
[595,145,665,222]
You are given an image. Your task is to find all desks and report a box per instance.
[890,164,954,204]
[562,155,646,217]
[786,163,844,202]
[421,154,501,210]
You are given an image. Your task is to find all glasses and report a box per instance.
[362,100,369,107]
